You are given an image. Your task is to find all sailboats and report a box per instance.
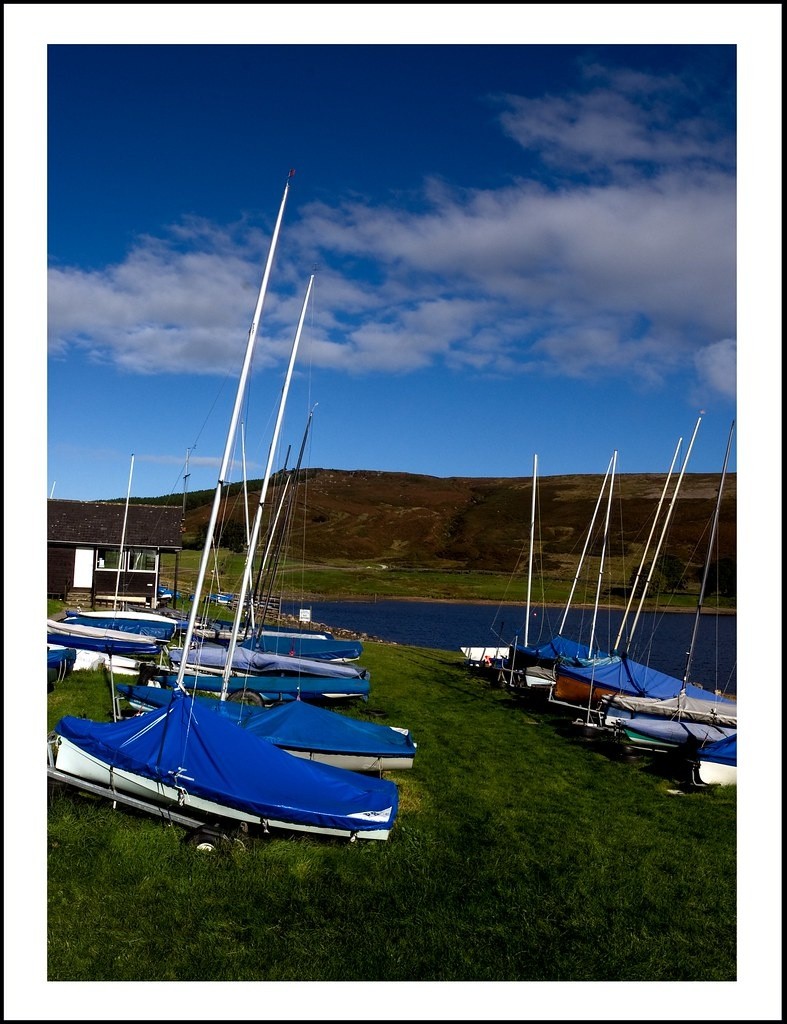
[46,405,369,714]
[108,272,415,771]
[48,169,401,845]
[507,409,735,792]
